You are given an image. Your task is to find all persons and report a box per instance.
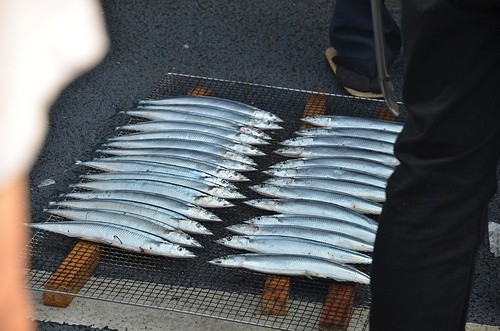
[368,0,500,331]
[325,0,402,99]
[1,0,113,330]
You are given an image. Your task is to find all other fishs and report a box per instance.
[22,98,404,286]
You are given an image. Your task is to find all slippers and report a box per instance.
[324,45,395,98]
